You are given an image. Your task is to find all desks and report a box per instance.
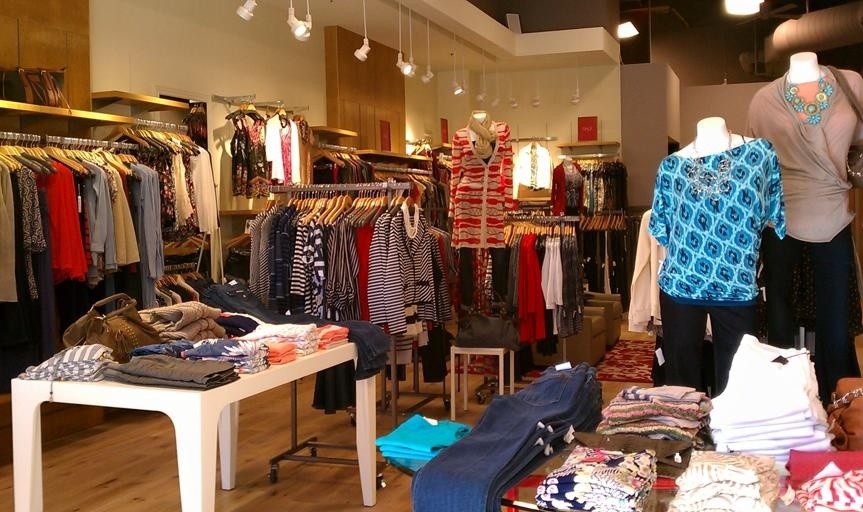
[9,335,379,511]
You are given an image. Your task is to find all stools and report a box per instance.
[450,345,515,422]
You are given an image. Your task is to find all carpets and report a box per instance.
[447,338,655,384]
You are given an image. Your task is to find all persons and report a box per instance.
[744,52,863,413]
[448,110,513,310]
[550,157,584,216]
[646,116,787,399]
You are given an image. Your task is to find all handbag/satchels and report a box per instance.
[85,292,162,365]
[453,288,520,349]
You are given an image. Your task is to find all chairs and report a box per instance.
[530,290,622,367]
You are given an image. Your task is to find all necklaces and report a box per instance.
[563,160,575,174]
[784,65,833,126]
[687,130,732,201]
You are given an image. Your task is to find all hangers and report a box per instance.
[517,136,549,155]
[0,118,210,185]
[501,208,628,250]
[181,93,313,135]
[250,182,452,245]
[310,142,449,191]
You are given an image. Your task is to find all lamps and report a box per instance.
[421,71,434,84]
[474,92,542,106]
[286,7,313,42]
[353,45,371,63]
[452,79,466,96]
[236,2,259,22]
[568,90,579,104]
[396,53,417,78]
